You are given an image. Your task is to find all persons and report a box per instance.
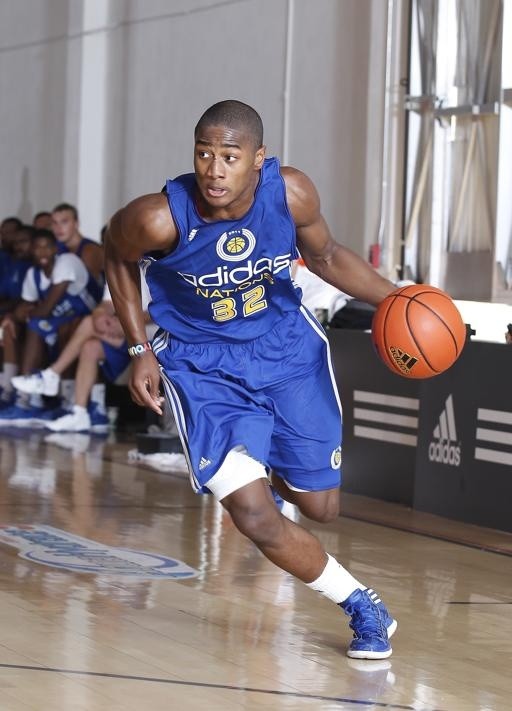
[0,203,160,436]
[101,101,402,660]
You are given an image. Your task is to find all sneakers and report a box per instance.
[11,370,60,397]
[0,398,109,436]
[337,587,398,660]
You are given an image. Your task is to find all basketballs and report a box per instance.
[372,284,467,381]
